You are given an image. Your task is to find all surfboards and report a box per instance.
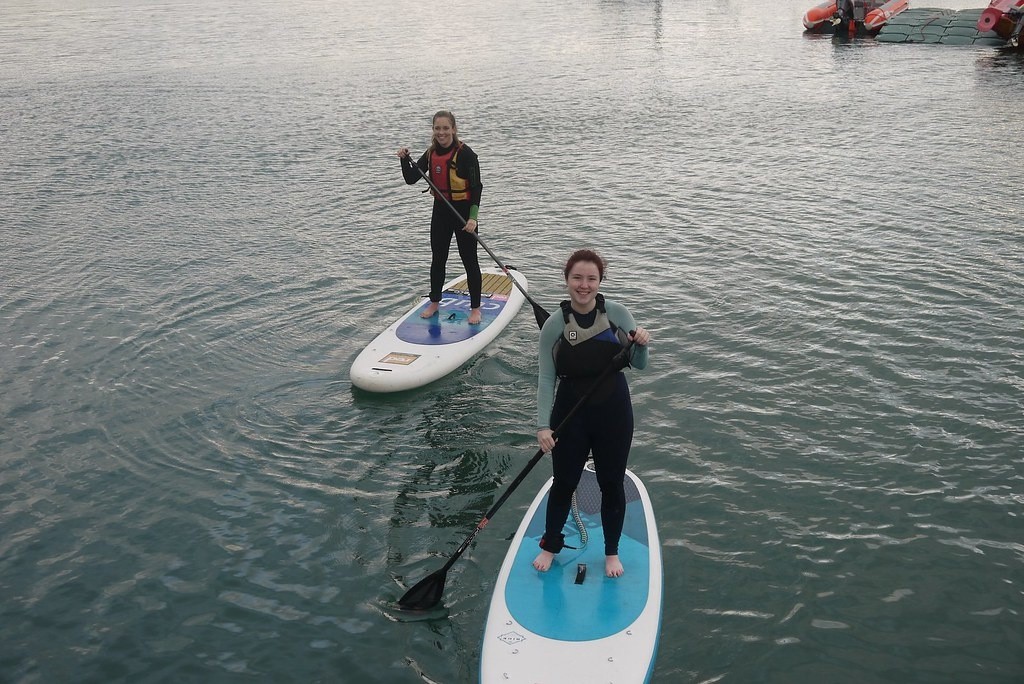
[350,265,528,394]
[479,458,664,684]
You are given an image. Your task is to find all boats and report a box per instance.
[975,0,1024,48]
[801,0,910,32]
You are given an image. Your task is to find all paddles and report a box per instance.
[405,149,551,331]
[397,330,637,610]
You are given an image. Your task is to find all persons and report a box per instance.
[532,249,651,578]
[396,110,483,324]
